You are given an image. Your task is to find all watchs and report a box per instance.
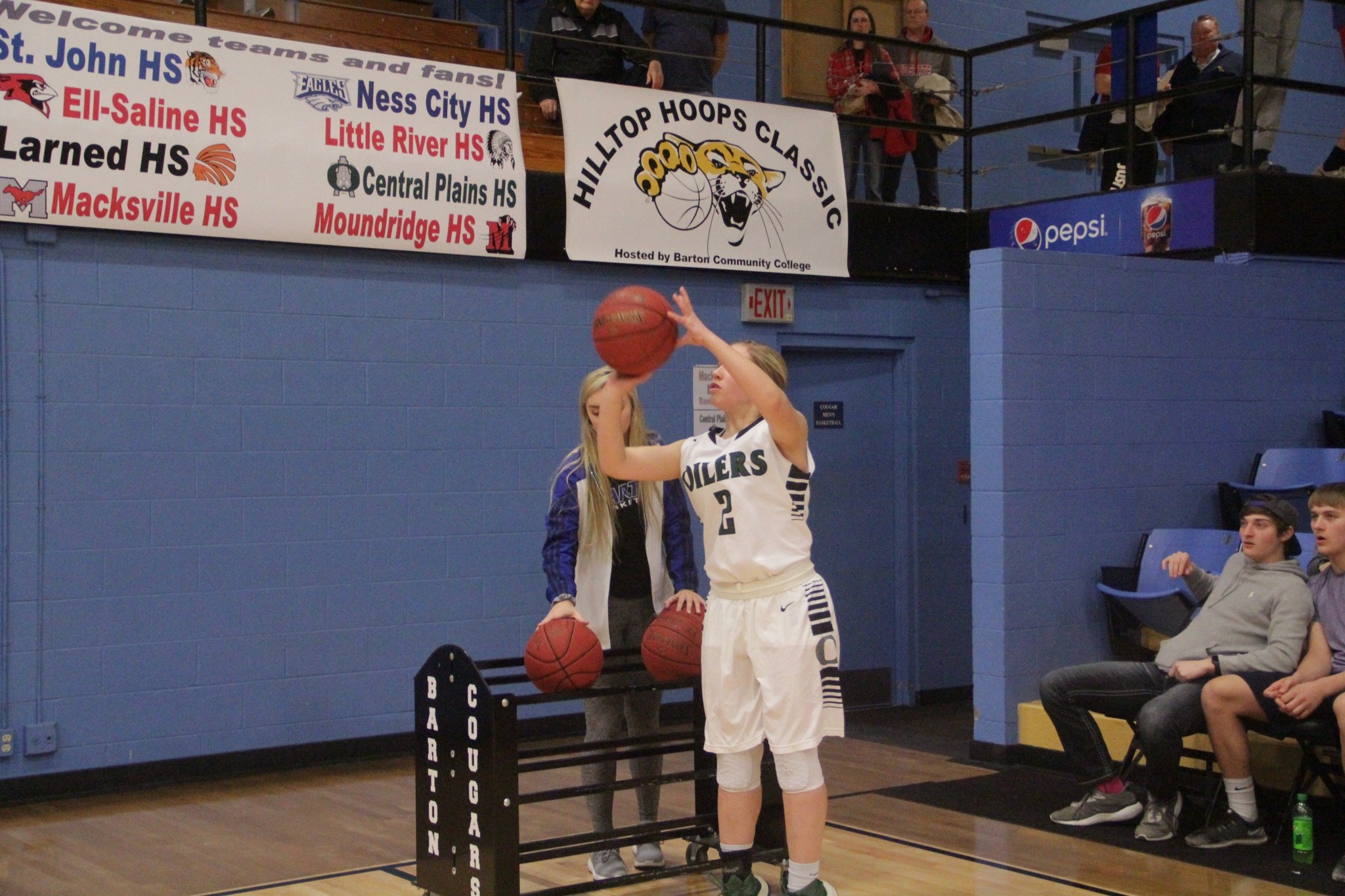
[1212,656,1221,676]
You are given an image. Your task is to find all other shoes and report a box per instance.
[1259,161,1287,173]
[1218,164,1248,172]
[1312,164,1345,179]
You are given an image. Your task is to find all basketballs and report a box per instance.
[640,599,705,682]
[591,284,679,377]
[523,618,605,693]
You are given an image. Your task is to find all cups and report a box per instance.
[1140,198,1172,253]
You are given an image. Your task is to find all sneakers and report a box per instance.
[632,841,665,866]
[1185,808,1268,849]
[1134,788,1182,841]
[1331,854,1345,882]
[780,870,837,896]
[706,870,771,896]
[1049,781,1143,826]
[588,847,628,880]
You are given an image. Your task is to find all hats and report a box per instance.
[1244,493,1302,556]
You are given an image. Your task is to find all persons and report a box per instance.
[641,0,728,98]
[1184,482,1345,881]
[525,0,664,135]
[1312,0,1345,177]
[826,6,902,203]
[882,0,958,207]
[596,285,845,896]
[1038,493,1315,840]
[541,364,707,880]
[1156,14,1244,181]
[1093,13,1174,191]
[1219,0,1303,175]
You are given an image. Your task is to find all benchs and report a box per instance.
[56,0,565,172]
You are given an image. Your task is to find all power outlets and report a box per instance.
[25,721,58,755]
[0,728,15,756]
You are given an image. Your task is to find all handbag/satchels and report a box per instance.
[839,84,865,115]
[1077,92,1111,150]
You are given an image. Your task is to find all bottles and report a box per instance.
[844,80,859,95]
[1290,792,1314,864]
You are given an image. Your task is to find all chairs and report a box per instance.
[1096,408,1345,862]
